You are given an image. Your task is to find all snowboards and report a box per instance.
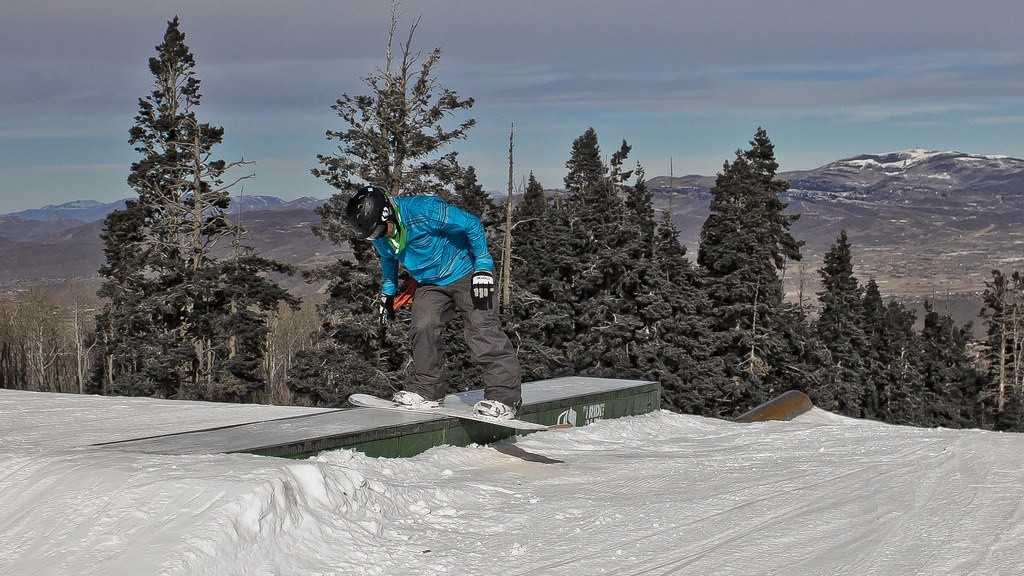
[347,392,573,430]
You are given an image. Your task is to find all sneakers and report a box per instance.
[392,391,446,408]
[472,400,519,420]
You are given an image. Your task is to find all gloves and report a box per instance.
[472,270,495,299]
[378,292,396,327]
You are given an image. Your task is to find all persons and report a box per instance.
[345,186,522,421]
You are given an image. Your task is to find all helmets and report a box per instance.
[346,186,401,241]
